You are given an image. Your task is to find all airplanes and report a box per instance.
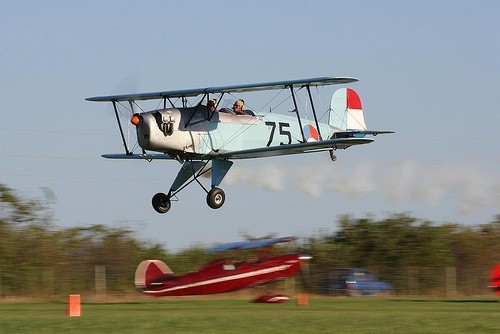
[131,235,315,299]
[85,75,396,215]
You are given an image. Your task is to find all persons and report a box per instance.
[232,99,248,115]
[206,98,219,112]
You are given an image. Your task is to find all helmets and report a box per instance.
[208,100,216,106]
[234,100,244,107]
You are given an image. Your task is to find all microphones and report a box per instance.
[232,107,235,109]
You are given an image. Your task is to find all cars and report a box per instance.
[319,267,393,298]
